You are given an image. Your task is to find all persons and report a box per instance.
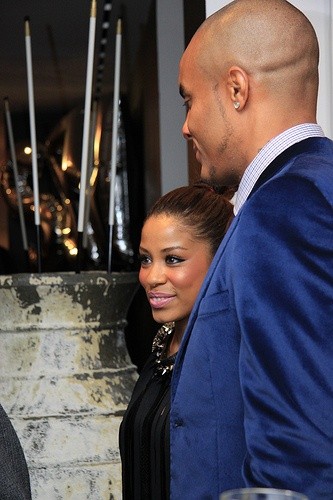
[118,180,236,500]
[168,0,333,500]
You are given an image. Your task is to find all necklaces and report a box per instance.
[152,322,178,375]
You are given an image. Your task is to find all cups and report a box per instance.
[220,488,308,500]
